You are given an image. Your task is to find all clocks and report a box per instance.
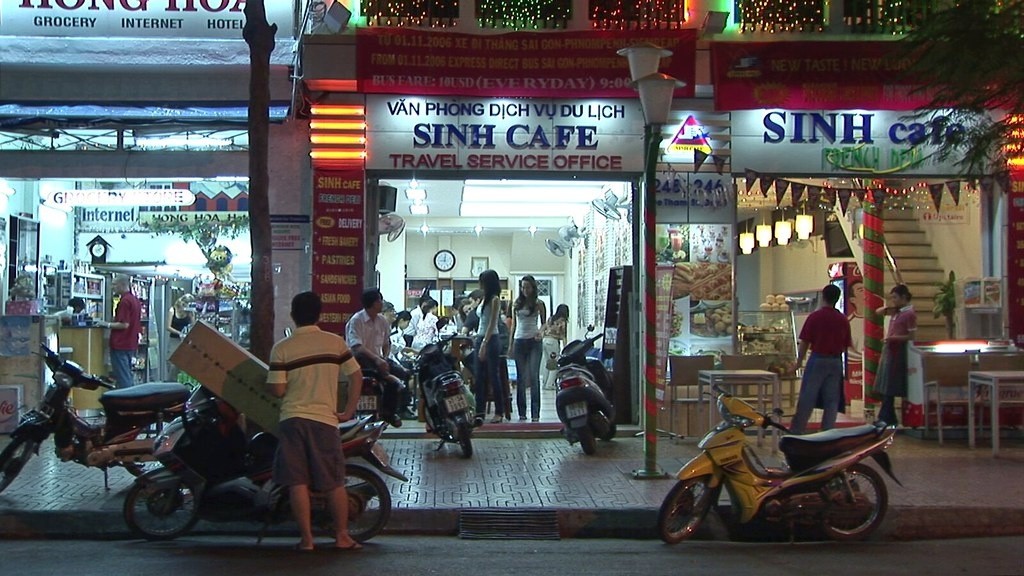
[434,250,456,272]
[86,235,112,264]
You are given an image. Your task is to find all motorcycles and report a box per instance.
[654,381,906,545]
[395,330,485,458]
[0,338,198,496]
[548,324,617,455]
[122,380,411,548]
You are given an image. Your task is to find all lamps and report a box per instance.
[740,203,814,254]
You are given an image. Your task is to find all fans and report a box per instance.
[545,225,588,259]
[592,190,632,223]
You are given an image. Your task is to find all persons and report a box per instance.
[872,285,917,427]
[92,274,141,389]
[54,297,86,316]
[473,269,504,424]
[381,290,513,395]
[507,275,548,421]
[845,281,864,364]
[259,292,366,551]
[785,285,853,437]
[541,304,569,389]
[344,286,406,429]
[165,293,196,382]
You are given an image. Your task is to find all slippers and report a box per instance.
[292,542,315,553]
[335,542,363,551]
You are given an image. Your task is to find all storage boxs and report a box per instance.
[0,384,24,433]
[168,319,283,438]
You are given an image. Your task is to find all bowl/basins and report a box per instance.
[690,300,701,312]
[701,300,725,309]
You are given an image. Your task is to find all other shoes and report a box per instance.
[872,419,888,427]
[475,417,483,424]
[532,418,539,422]
[383,409,402,428]
[491,415,502,423]
[391,377,406,396]
[518,417,527,422]
[541,385,555,390]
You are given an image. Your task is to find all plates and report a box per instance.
[689,313,734,340]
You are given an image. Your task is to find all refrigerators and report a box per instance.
[901,338,1024,427]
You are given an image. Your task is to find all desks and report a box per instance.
[698,370,780,452]
[968,371,1024,459]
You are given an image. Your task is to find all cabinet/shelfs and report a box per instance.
[737,310,797,370]
[40,263,151,417]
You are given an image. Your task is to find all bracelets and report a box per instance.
[108,322,111,328]
[482,339,489,344]
